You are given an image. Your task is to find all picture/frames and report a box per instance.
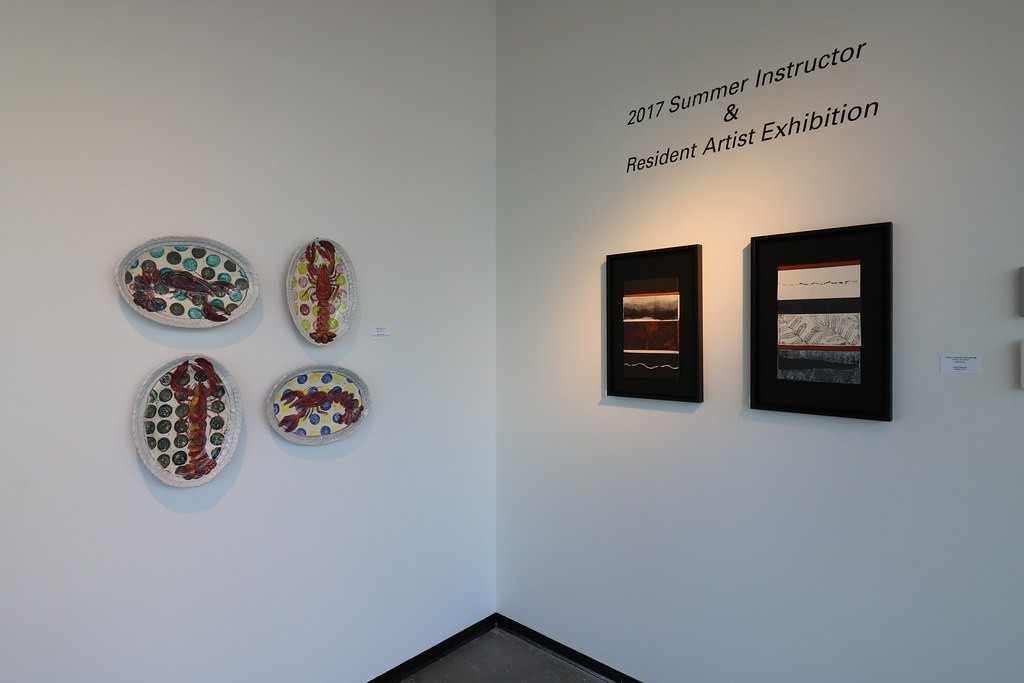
[750,221,894,421]
[115,236,260,328]
[286,237,357,347]
[605,244,702,405]
[266,364,371,446]
[132,355,243,487]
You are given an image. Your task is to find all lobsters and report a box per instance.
[278,390,362,432]
[133,269,237,322]
[170,357,218,480]
[305,240,340,344]
